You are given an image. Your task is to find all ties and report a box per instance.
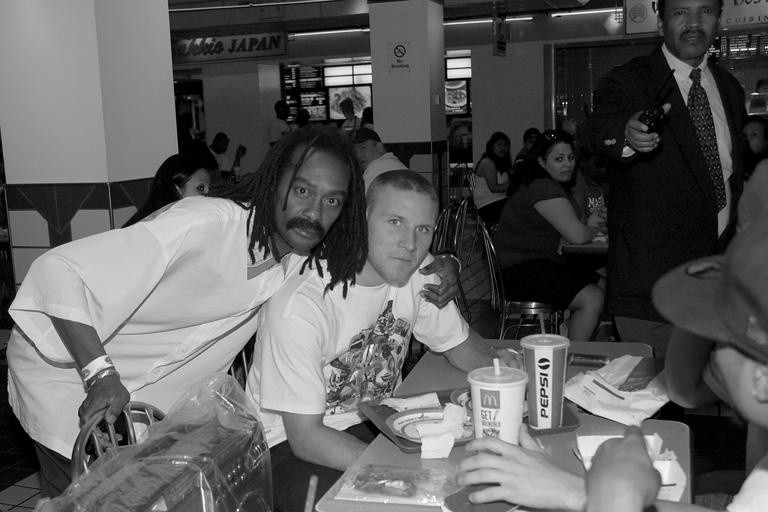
[686,69,727,214]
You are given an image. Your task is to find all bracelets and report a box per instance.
[451,255,461,272]
[80,355,118,391]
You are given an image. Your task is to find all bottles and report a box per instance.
[232,156,240,183]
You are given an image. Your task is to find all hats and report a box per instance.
[652,210,768,363]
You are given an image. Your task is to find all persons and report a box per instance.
[349,127,410,191]
[742,116,768,159]
[340,99,373,131]
[494,128,604,340]
[123,153,214,229]
[243,169,524,512]
[207,134,247,174]
[268,102,312,148]
[474,129,541,226]
[5,125,461,501]
[578,0,757,367]
[457,159,768,512]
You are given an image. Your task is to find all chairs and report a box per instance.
[474,212,559,338]
[426,158,483,268]
[68,399,166,488]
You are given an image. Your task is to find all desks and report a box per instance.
[556,235,610,255]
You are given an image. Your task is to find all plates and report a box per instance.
[386,408,476,445]
[450,387,530,418]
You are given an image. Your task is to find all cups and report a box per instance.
[519,334,572,431]
[466,365,529,450]
